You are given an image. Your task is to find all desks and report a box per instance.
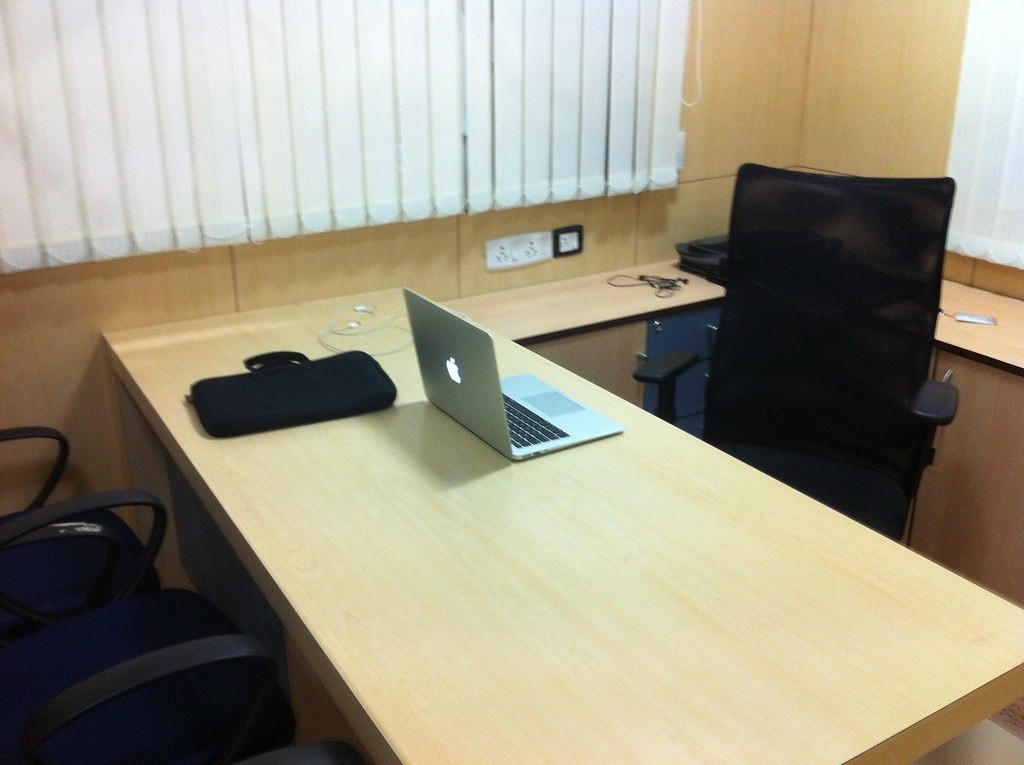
[102,288,1024,765]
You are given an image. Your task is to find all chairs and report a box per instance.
[632,163,958,542]
[0,426,295,765]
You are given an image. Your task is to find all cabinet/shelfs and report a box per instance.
[436,251,1024,740]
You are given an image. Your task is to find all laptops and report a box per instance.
[402,286,625,461]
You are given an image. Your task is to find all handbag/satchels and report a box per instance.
[189,350,398,437]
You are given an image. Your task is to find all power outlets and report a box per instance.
[484,232,552,270]
